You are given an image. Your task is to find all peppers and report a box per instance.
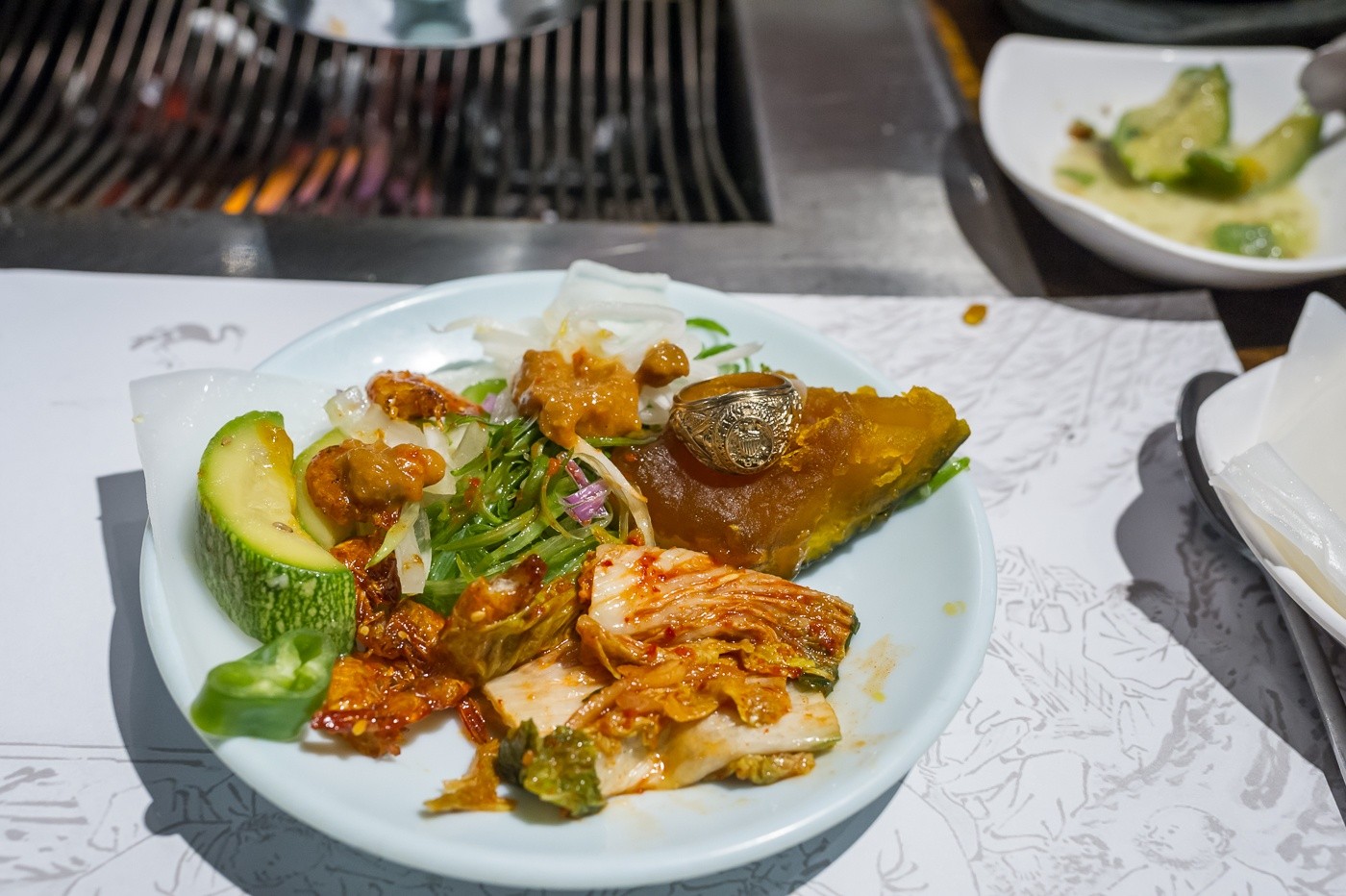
[190,626,338,741]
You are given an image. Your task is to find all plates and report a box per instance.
[139,270,996,891]
[978,32,1346,288]
[1196,352,1346,646]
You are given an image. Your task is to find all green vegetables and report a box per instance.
[410,322,769,609]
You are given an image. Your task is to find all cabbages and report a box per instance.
[307,533,856,816]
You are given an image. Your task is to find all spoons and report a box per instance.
[1175,370,1346,785]
